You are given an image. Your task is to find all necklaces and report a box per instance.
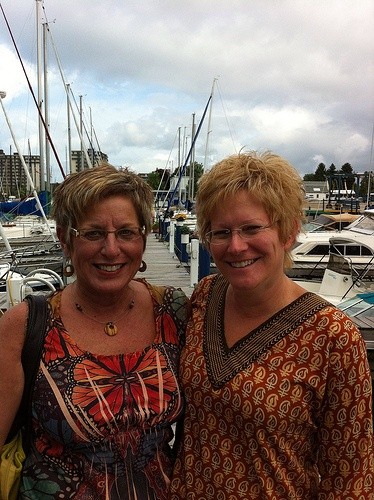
[71,280,136,336]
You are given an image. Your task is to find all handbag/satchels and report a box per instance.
[1,295,48,499]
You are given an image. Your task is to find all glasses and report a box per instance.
[204,221,277,246]
[68,226,146,244]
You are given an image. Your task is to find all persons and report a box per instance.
[167,149,373,499]
[0,163,191,500]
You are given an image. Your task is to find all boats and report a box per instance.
[1,1,373,358]
